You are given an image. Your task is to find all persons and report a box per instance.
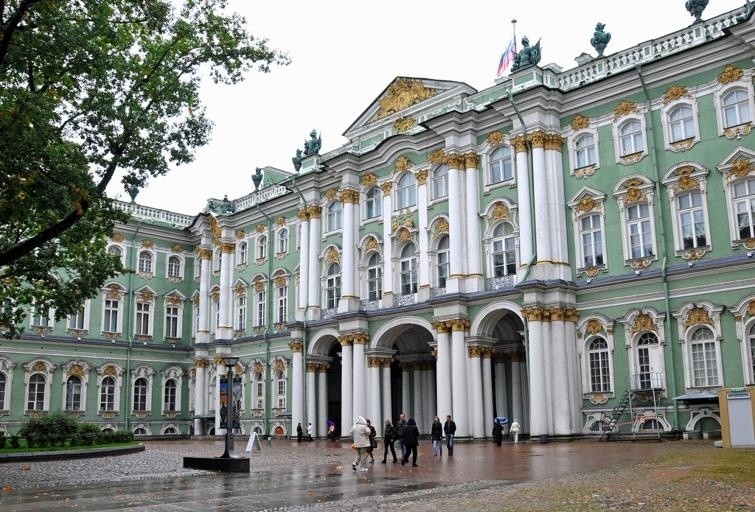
[510,419,520,446]
[513,35,537,69]
[365,419,376,463]
[328,424,335,442]
[350,416,371,472]
[444,415,456,456]
[492,420,503,447]
[431,416,442,456]
[304,129,319,156]
[297,423,303,442]
[381,414,420,467]
[308,422,314,441]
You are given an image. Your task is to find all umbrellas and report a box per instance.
[495,416,510,424]
[327,420,335,426]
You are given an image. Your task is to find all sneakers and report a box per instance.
[351,459,418,473]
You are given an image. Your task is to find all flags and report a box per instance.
[496,37,514,78]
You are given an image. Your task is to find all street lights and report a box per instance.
[219,353,243,457]
